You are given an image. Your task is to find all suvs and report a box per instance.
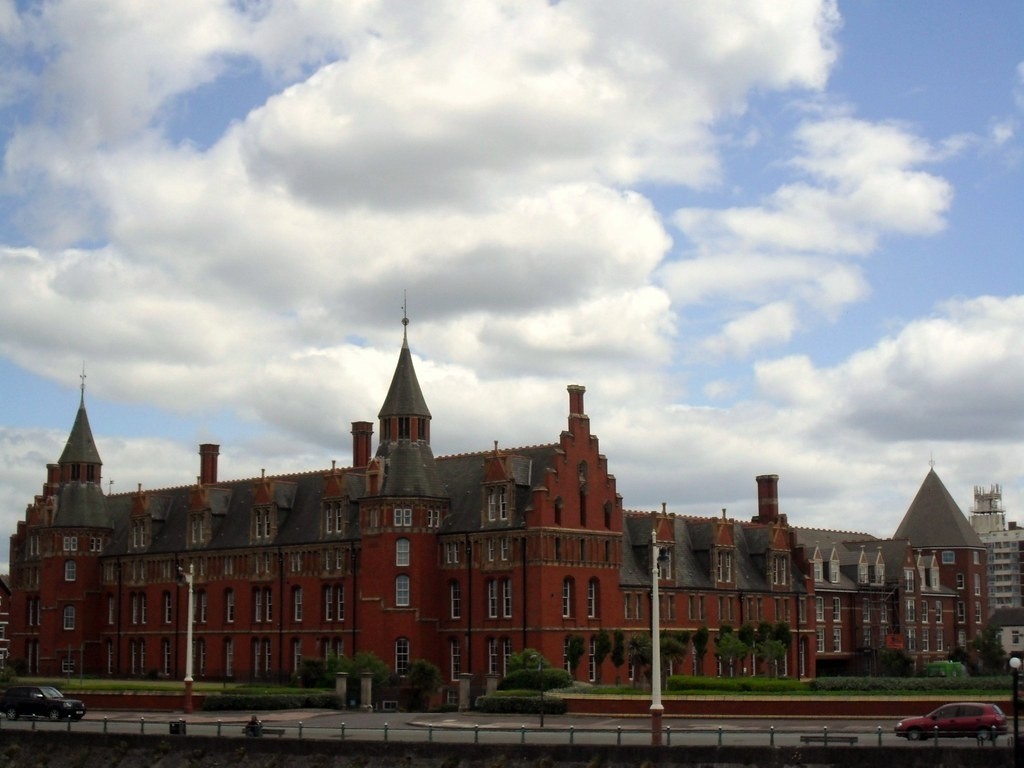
[895,701,1008,743]
[0,686,87,722]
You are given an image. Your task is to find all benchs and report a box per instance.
[799,735,858,746]
[242,727,286,737]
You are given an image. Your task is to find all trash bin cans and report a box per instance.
[168,719,187,736]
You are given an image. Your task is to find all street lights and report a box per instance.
[177,564,195,714]
[651,529,670,746]
[1010,657,1022,749]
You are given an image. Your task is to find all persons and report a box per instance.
[245,714,264,737]
